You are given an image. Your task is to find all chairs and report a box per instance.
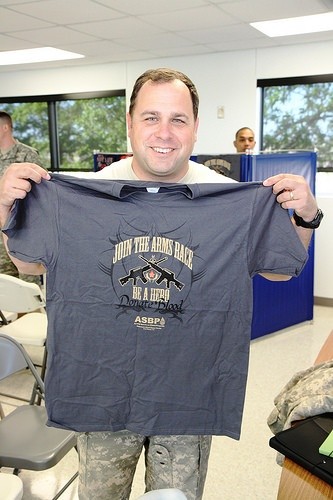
[0,233,79,500]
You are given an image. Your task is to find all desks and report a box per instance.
[269,328,333,500]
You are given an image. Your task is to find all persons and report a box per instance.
[0,112,47,319]
[234,127,256,154]
[0,67,324,500]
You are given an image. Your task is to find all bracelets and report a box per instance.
[293,209,323,229]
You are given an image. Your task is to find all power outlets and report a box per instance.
[218,106,224,119]
